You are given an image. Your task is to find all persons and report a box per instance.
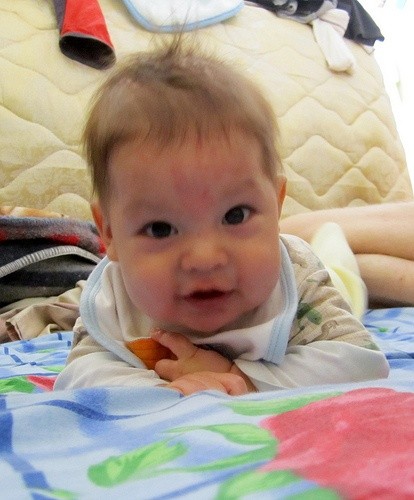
[53,15,390,396]
